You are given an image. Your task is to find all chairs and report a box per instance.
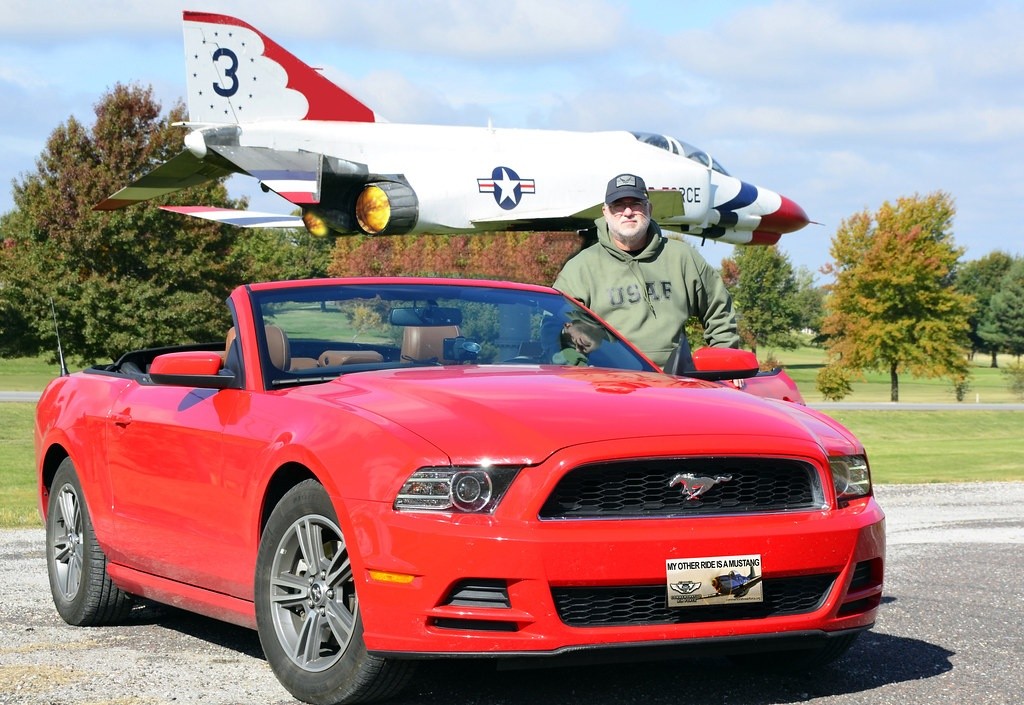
[223,324,462,372]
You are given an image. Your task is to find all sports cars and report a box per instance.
[34,277,888,705]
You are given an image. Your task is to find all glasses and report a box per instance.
[604,201,649,216]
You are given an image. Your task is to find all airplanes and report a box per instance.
[92,12,814,249]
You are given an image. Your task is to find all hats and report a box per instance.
[605,174,649,204]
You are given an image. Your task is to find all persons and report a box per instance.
[560,310,617,356]
[540,173,745,388]
